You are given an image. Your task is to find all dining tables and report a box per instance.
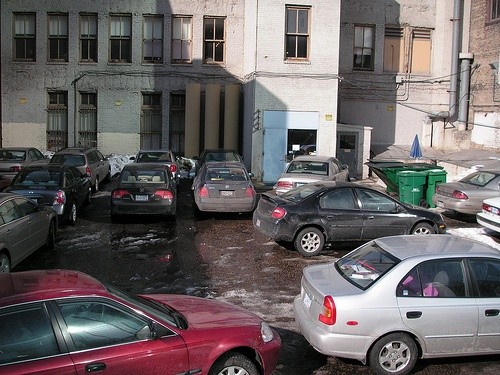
[124,171,131,181]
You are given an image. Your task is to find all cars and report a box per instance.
[110,161,178,223]
[0,267,283,375]
[273,155,352,196]
[128,148,184,194]
[0,193,59,275]
[191,148,257,214]
[0,147,49,192]
[476,195,500,239]
[292,233,500,375]
[431,169,500,224]
[252,180,448,257]
[3,162,93,227]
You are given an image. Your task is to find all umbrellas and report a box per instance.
[409,133,423,158]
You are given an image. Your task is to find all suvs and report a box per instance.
[49,145,113,194]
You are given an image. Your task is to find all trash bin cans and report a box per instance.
[365,158,447,210]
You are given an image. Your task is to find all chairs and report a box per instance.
[433,271,456,297]
[155,172,164,181]
[153,176,161,181]
[6,153,13,159]
[236,176,244,181]
[140,155,149,162]
[210,173,218,178]
[128,176,135,181]
